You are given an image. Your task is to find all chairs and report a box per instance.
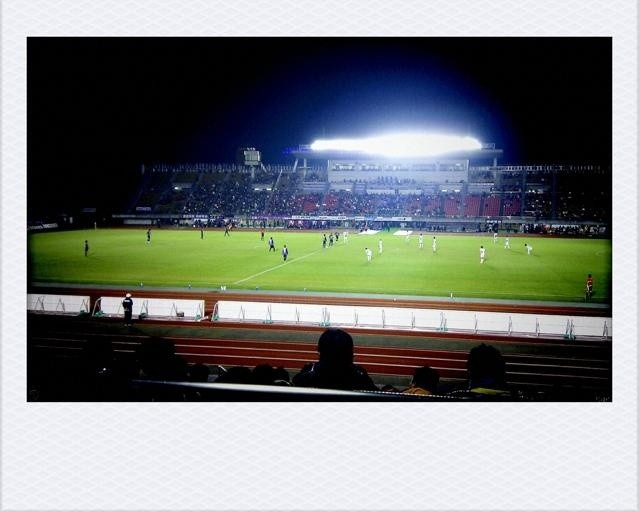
[299,190,525,218]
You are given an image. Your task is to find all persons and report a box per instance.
[282,245,288,261]
[365,247,372,261]
[503,235,510,249]
[128,165,609,238]
[328,233,334,248]
[27,327,515,403]
[145,228,151,247]
[85,240,88,256]
[418,233,424,249]
[432,236,436,252]
[321,233,326,248]
[479,245,485,264]
[584,273,594,299]
[377,239,383,255]
[268,237,275,252]
[524,243,533,257]
